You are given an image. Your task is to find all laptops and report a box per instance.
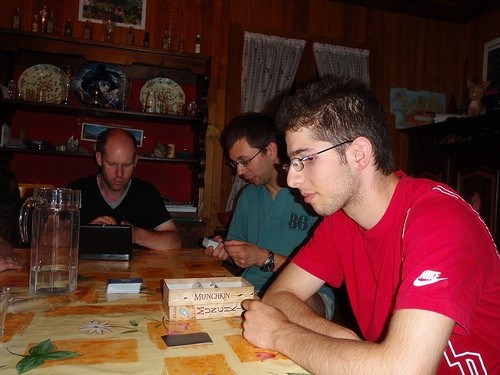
[78,224,133,261]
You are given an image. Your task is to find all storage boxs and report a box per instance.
[163,277,255,321]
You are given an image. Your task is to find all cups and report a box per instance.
[36,89,46,104]
[19,186,81,297]
[26,87,36,103]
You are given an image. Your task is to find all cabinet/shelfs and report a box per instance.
[400,112,500,254]
[0,28,212,251]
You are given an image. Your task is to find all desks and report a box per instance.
[0,248,312,375]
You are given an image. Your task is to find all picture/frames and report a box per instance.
[78,0,147,30]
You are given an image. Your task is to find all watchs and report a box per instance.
[260,250,275,272]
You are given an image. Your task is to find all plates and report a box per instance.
[73,63,129,109]
[17,64,71,105]
[139,77,186,116]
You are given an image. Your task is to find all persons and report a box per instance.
[241,75,500,375]
[0,168,24,274]
[43,128,182,250]
[203,113,335,321]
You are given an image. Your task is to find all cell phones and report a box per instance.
[160,333,213,347]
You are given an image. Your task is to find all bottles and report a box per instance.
[144,91,156,114]
[64,17,72,36]
[143,32,150,48]
[30,15,39,33]
[11,7,21,30]
[162,31,169,51]
[194,34,201,54]
[103,22,114,43]
[127,26,135,45]
[46,13,54,34]
[178,35,184,53]
[7,80,16,100]
[84,20,91,40]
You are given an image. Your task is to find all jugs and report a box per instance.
[78,90,114,108]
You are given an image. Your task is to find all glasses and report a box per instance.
[283,139,355,176]
[229,143,271,169]
